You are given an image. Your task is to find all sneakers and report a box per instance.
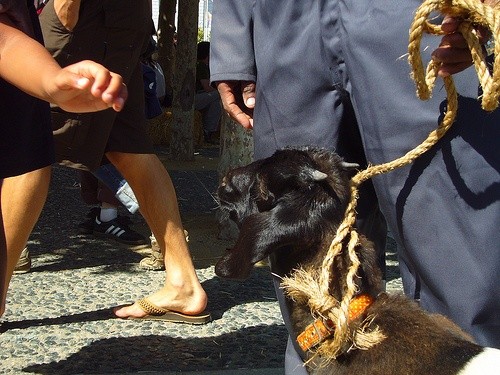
[139,229,190,270]
[92,215,147,245]
[12,246,31,274]
[78,206,101,234]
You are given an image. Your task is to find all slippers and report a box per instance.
[110,296,212,324]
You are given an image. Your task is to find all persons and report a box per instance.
[195,42,221,144]
[209,0,500,375]
[0,0,211,325]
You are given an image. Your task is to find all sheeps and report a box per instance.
[215,144,500,375]
